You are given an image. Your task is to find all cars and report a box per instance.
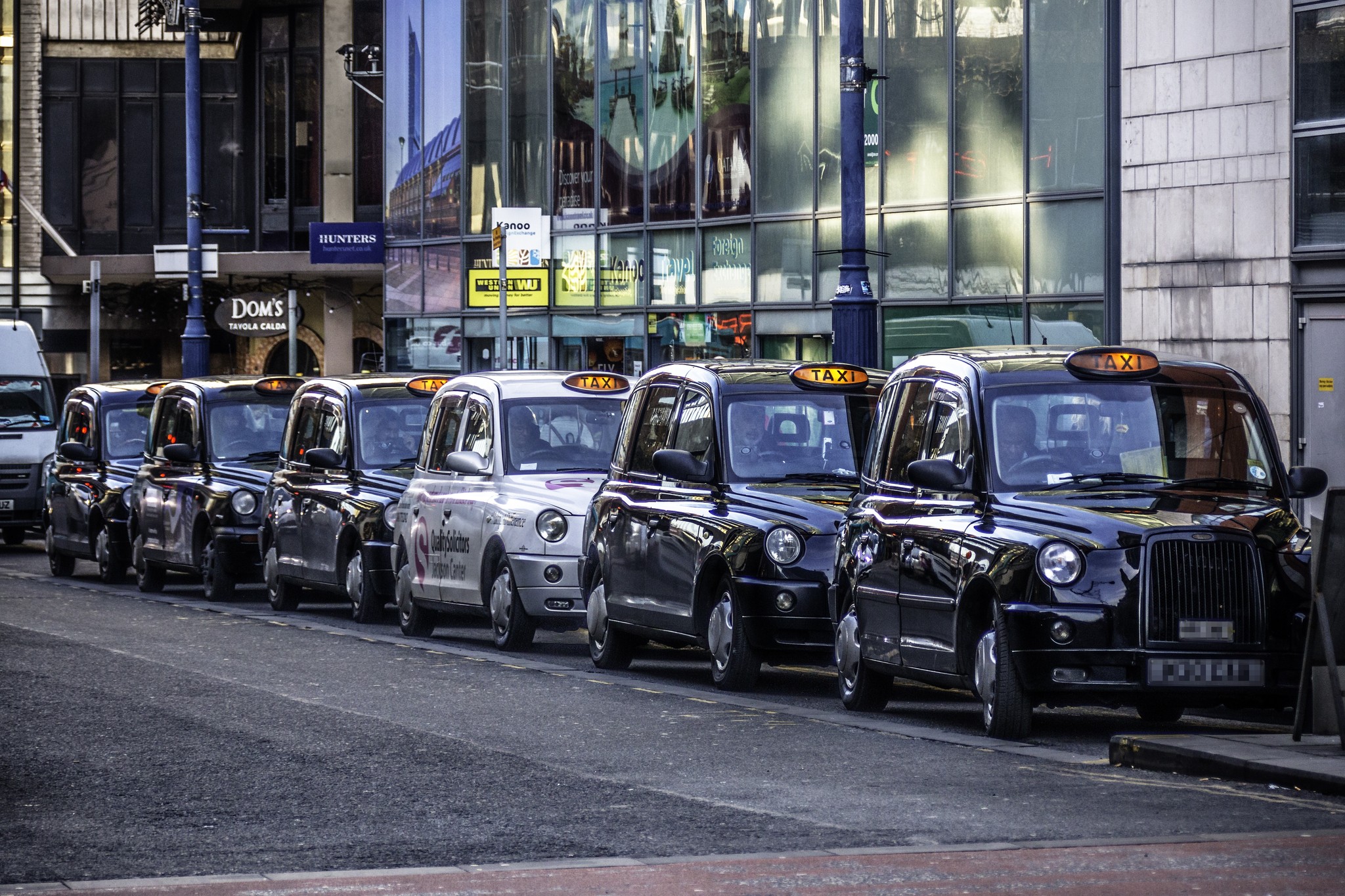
[390,371,639,654]
[577,359,894,693]
[125,374,320,601]
[41,379,182,585]
[257,374,465,623]
[821,341,1329,741]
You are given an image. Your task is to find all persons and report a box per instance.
[211,403,257,449]
[363,408,416,459]
[997,418,1040,474]
[1065,396,1110,449]
[509,405,552,462]
[728,401,781,463]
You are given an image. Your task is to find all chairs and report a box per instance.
[399,408,429,435]
[996,405,1040,455]
[1046,404,1098,448]
[769,413,811,445]
[118,411,145,432]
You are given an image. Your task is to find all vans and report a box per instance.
[0,317,63,545]
